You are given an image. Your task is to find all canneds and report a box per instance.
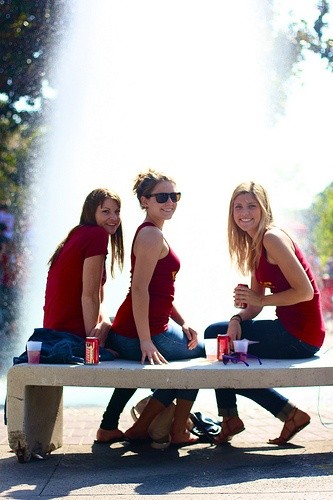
[217,334,230,361]
[234,284,248,308]
[85,336,99,365]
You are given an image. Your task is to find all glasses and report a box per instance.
[148,193,181,203]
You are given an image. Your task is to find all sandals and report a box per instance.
[94,430,127,444]
[268,408,310,443]
[214,428,245,444]
[172,436,202,447]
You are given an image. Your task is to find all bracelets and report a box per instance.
[229,314,242,324]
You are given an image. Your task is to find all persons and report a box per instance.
[204,182,326,444]
[43,189,140,444]
[108,168,207,445]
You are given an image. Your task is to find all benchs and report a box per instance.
[6,354,333,463]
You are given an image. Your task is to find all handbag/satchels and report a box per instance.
[135,395,195,440]
[192,412,223,440]
